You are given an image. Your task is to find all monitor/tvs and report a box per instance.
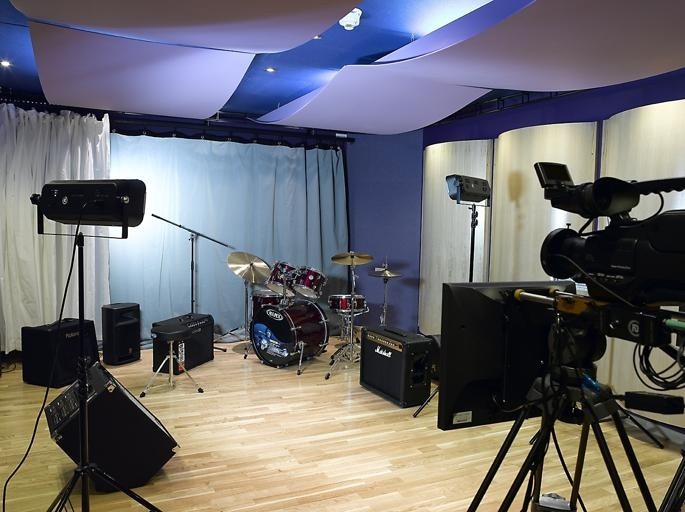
[437,281,577,431]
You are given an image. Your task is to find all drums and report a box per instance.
[249,300,329,369]
[329,293,366,313]
[292,266,328,299]
[264,259,299,296]
[251,289,287,319]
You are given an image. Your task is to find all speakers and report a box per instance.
[18,317,100,388]
[151,311,214,373]
[359,327,433,409]
[102,303,140,366]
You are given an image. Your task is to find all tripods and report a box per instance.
[325,265,389,380]
[412,206,478,418]
[140,340,204,397]
[46,233,164,512]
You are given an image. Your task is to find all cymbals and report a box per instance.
[369,269,402,278]
[331,253,372,265]
[228,252,271,284]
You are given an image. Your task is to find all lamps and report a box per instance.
[339,8,362,32]
